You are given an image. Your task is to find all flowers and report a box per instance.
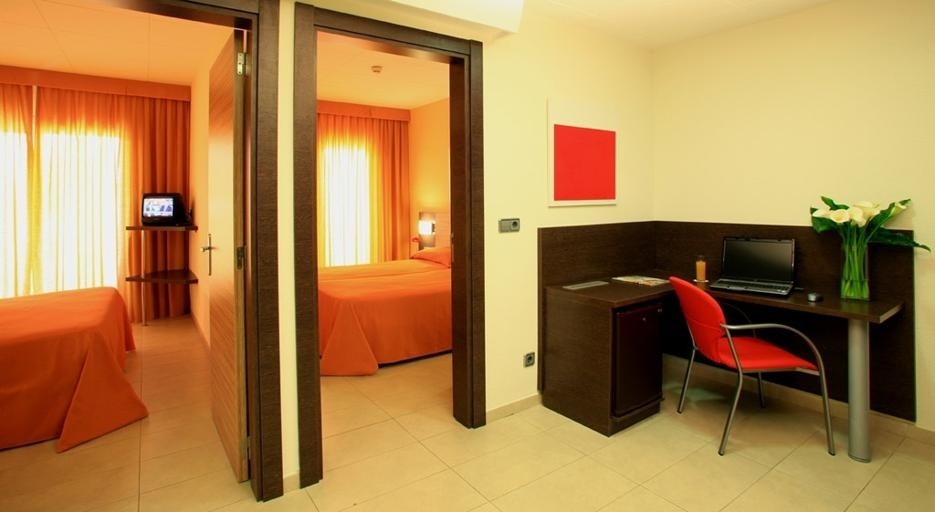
[809,194,931,299]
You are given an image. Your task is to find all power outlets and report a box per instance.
[499,218,520,232]
[524,352,535,367]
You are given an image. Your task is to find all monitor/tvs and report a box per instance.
[141,192,182,225]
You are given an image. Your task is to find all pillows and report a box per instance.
[410,245,450,266]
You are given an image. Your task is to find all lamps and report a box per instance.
[417,210,435,243]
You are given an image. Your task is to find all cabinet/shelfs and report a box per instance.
[125,225,197,328]
[540,294,663,438]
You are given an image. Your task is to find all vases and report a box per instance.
[836,241,872,301]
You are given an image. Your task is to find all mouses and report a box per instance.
[807,291,824,302]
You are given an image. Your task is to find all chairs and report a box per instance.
[668,275,837,456]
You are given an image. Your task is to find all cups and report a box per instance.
[695,255,706,282]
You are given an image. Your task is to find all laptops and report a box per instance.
[709,236,796,298]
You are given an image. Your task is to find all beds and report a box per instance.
[1,286,150,452]
[317,213,452,377]
